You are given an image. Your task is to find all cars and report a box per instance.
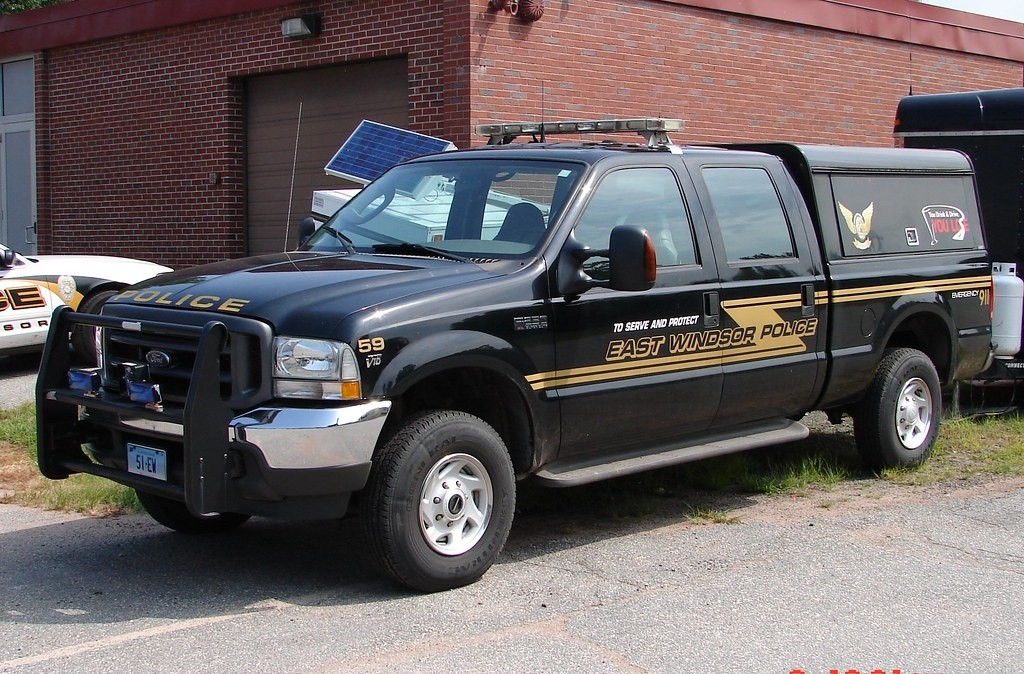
[0,243,173,359]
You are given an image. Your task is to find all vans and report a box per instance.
[892,87,1023,388]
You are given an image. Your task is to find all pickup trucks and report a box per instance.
[32,117,999,594]
